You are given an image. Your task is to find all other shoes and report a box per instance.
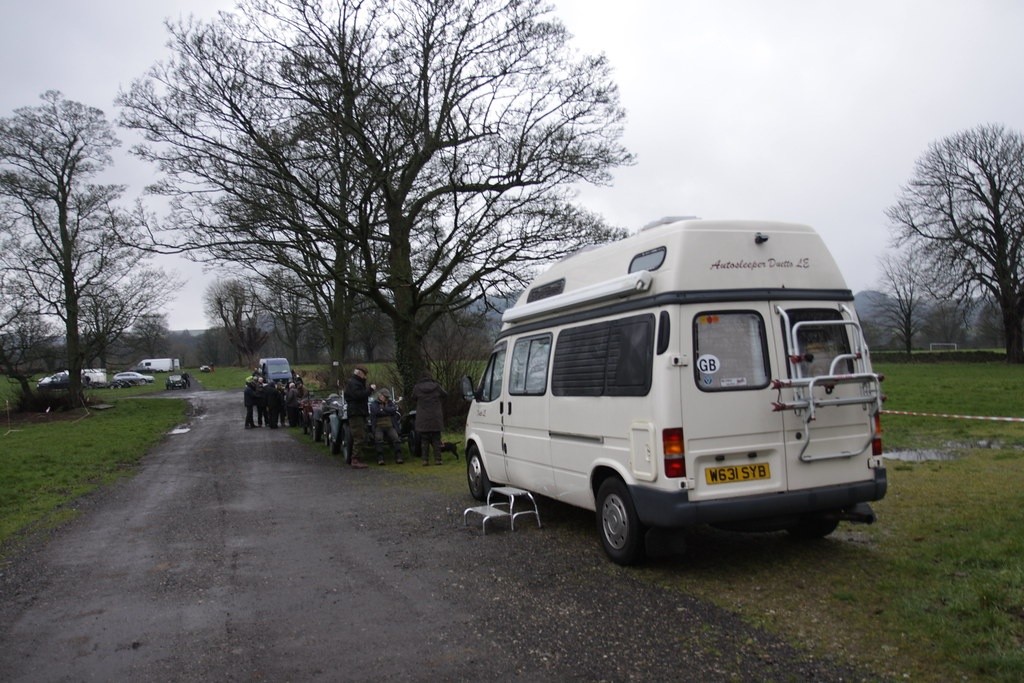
[351,463,368,468]
[422,461,430,466]
[396,458,404,463]
[435,460,443,465]
[378,460,385,465]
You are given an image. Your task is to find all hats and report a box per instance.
[356,367,368,375]
[380,388,391,396]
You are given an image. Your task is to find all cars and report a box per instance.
[36,368,107,389]
[108,372,155,387]
[165,375,186,390]
[200,365,210,372]
[297,384,423,464]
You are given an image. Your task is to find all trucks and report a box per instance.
[137,358,179,373]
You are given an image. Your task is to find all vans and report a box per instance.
[259,357,292,383]
[461,214,888,566]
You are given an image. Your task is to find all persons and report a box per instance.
[411,370,448,466]
[343,364,376,468]
[181,371,191,387]
[244,367,308,429]
[210,362,214,371]
[370,388,404,466]
[86,376,90,390]
